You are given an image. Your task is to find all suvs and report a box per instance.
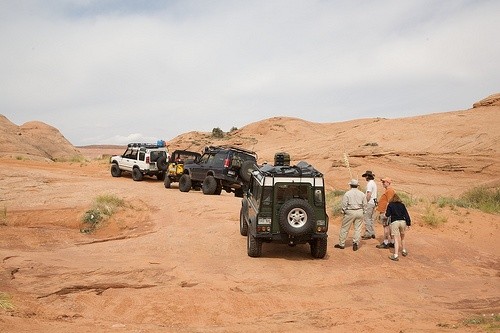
[109,143,170,181]
[164,149,202,189]
[178,145,258,198]
[239,161,329,258]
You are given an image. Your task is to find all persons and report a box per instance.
[361,171,378,239]
[335,179,367,251]
[383,193,411,261]
[375,177,395,249]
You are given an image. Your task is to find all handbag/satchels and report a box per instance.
[382,217,391,226]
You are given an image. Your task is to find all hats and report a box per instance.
[348,179,360,186]
[381,177,391,184]
[362,170,375,177]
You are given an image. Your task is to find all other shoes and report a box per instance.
[361,235,375,239]
[389,242,394,247]
[353,243,357,251]
[388,255,399,261]
[402,248,408,256]
[376,242,390,249]
[335,244,344,249]
